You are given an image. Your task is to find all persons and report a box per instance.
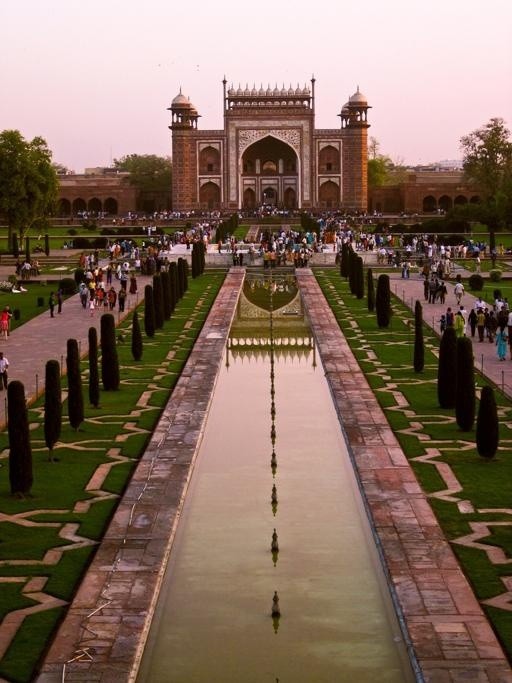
[218,220,503,279]
[15,259,40,279]
[237,200,383,219]
[3,303,13,336]
[49,235,173,317]
[423,276,511,360]
[73,205,223,248]
[0,307,9,340]
[0,351,10,391]
